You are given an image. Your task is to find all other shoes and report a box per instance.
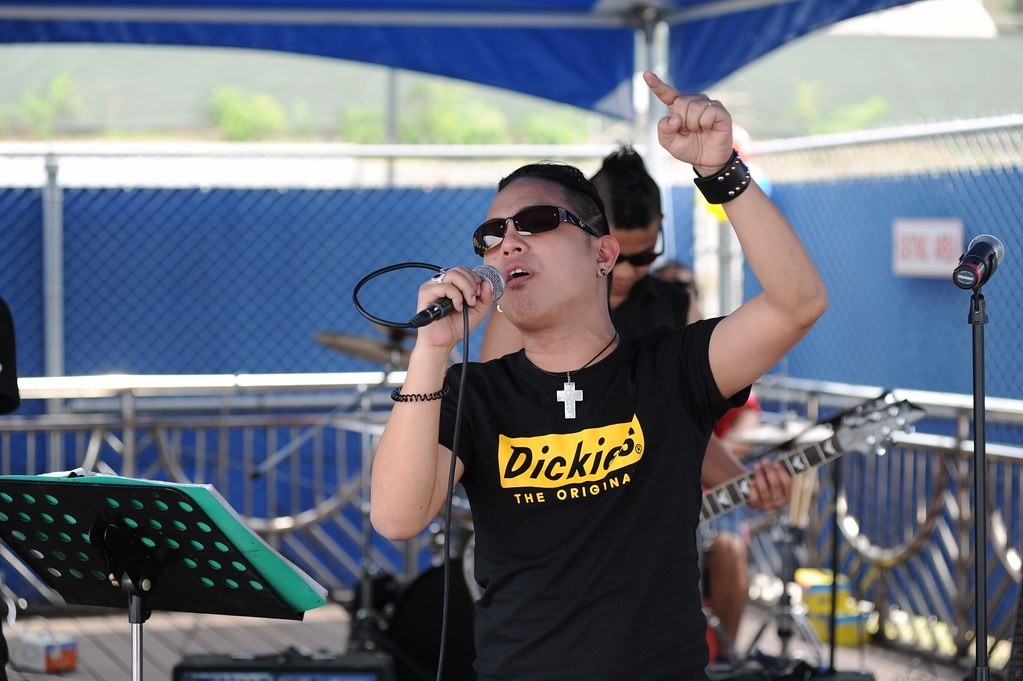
[710,654,732,671]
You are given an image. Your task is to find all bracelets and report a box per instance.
[391,383,450,402]
[692,150,751,205]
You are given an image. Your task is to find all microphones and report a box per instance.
[953,234,1005,290]
[409,265,507,328]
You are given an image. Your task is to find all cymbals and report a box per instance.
[312,328,417,371]
[729,416,834,445]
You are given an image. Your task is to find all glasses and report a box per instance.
[473,205,599,257]
[615,224,664,266]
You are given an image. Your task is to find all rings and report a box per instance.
[704,97,711,105]
[432,273,445,283]
[772,492,783,500]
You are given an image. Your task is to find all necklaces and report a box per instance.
[523,328,621,383]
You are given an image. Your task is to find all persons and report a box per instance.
[480,143,791,660]
[371,71,829,681]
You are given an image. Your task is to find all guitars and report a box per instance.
[458,387,930,607]
